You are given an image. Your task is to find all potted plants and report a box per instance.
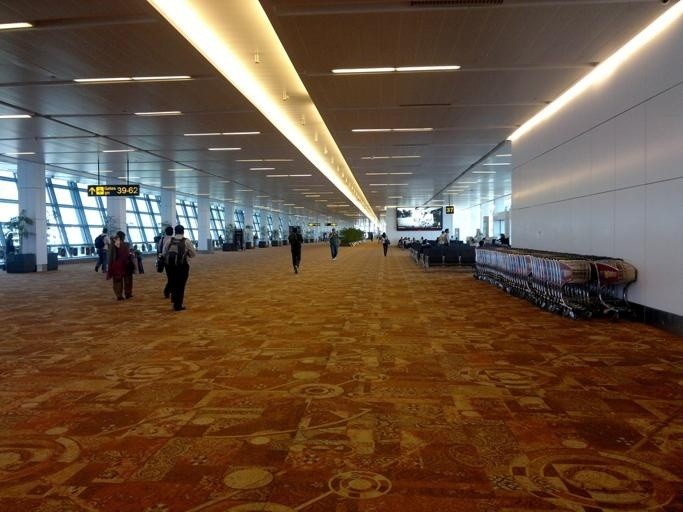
[220,223,366,253]
[2,210,36,272]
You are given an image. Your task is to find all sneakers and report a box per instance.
[171,300,185,311]
[116,293,135,301]
[95,265,99,272]
[164,291,170,299]
[294,265,298,273]
[102,269,107,273]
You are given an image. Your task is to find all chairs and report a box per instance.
[405,236,477,273]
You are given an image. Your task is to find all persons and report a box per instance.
[94,227,108,273]
[328,228,339,261]
[156,227,173,299]
[287,226,303,274]
[443,228,449,246]
[162,224,197,311]
[490,238,498,249]
[438,230,444,244]
[397,236,423,247]
[380,232,389,257]
[109,231,133,300]
[4,232,14,260]
[477,240,486,250]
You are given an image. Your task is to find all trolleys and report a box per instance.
[472,242,639,323]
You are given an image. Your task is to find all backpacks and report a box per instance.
[164,237,188,267]
[95,233,107,249]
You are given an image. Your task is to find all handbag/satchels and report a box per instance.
[156,255,165,273]
[126,261,135,274]
[385,240,390,244]
